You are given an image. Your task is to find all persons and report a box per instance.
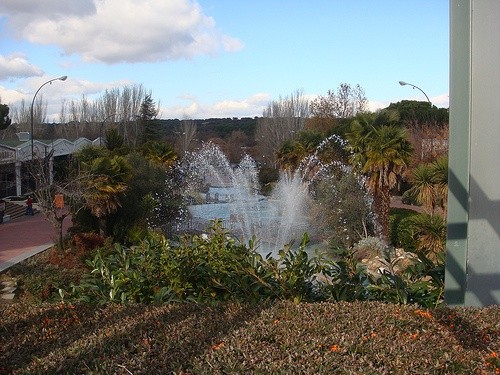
[26,196,34,216]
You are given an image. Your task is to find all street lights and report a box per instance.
[30,75,67,197]
